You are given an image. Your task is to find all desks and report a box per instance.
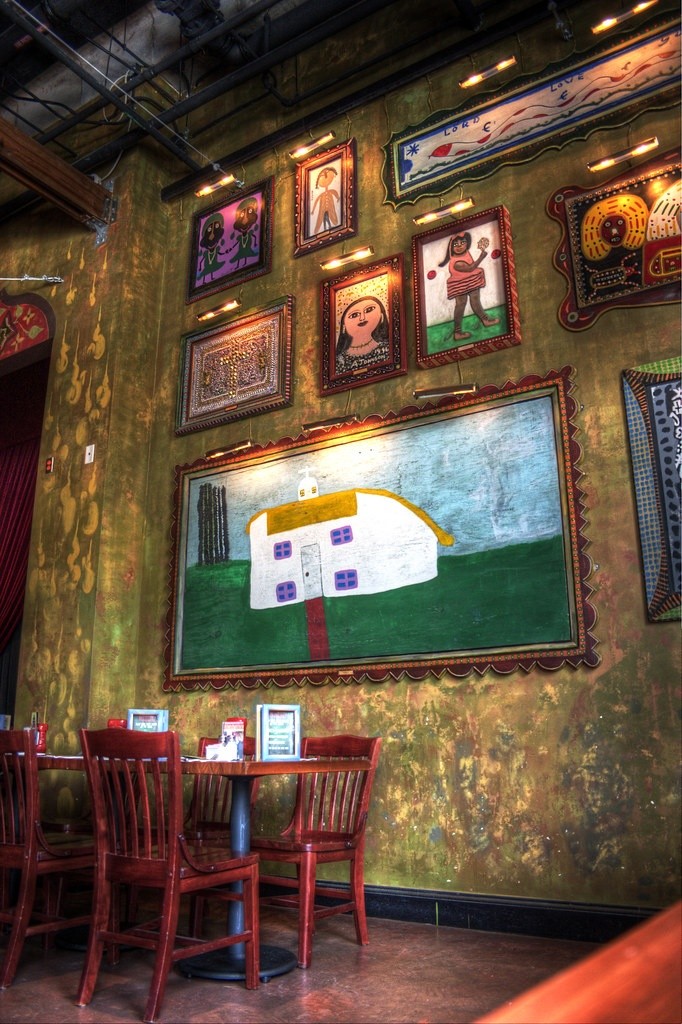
[0,750,373,983]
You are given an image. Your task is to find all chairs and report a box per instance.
[188,734,383,969]
[126,735,261,935]
[76,728,260,1024]
[0,727,121,990]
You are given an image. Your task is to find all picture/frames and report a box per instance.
[174,294,296,437]
[184,175,274,305]
[292,137,357,260]
[410,204,522,371]
[379,9,682,213]
[161,365,603,693]
[319,252,408,399]
[545,144,682,333]
[621,355,682,622]
